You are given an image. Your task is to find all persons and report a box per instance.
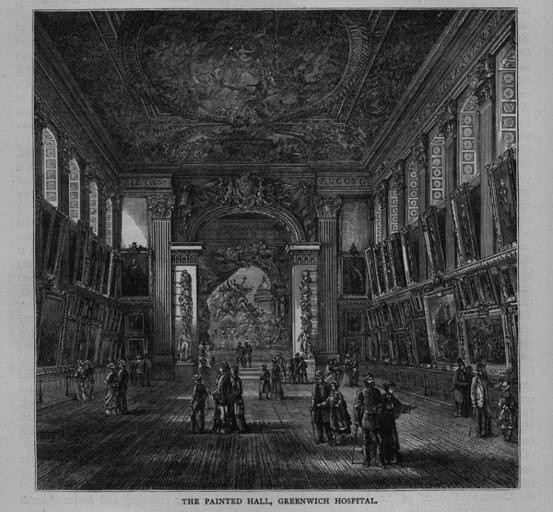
[61,340,518,466]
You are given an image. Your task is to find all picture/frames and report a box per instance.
[338,148,516,388]
[36,188,156,373]
